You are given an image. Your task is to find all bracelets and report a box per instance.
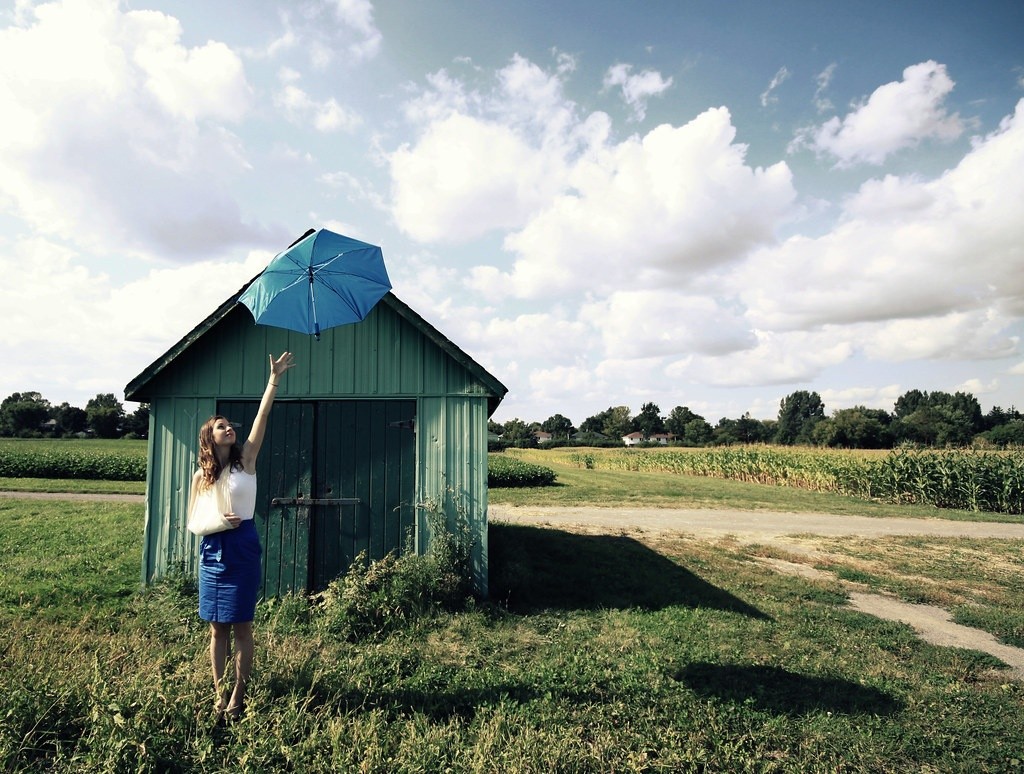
[268,381,279,388]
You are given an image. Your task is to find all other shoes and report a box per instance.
[229,690,245,720]
[213,690,230,719]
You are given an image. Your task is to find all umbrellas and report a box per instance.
[234,228,392,338]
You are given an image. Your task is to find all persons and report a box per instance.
[186,351,298,721]
